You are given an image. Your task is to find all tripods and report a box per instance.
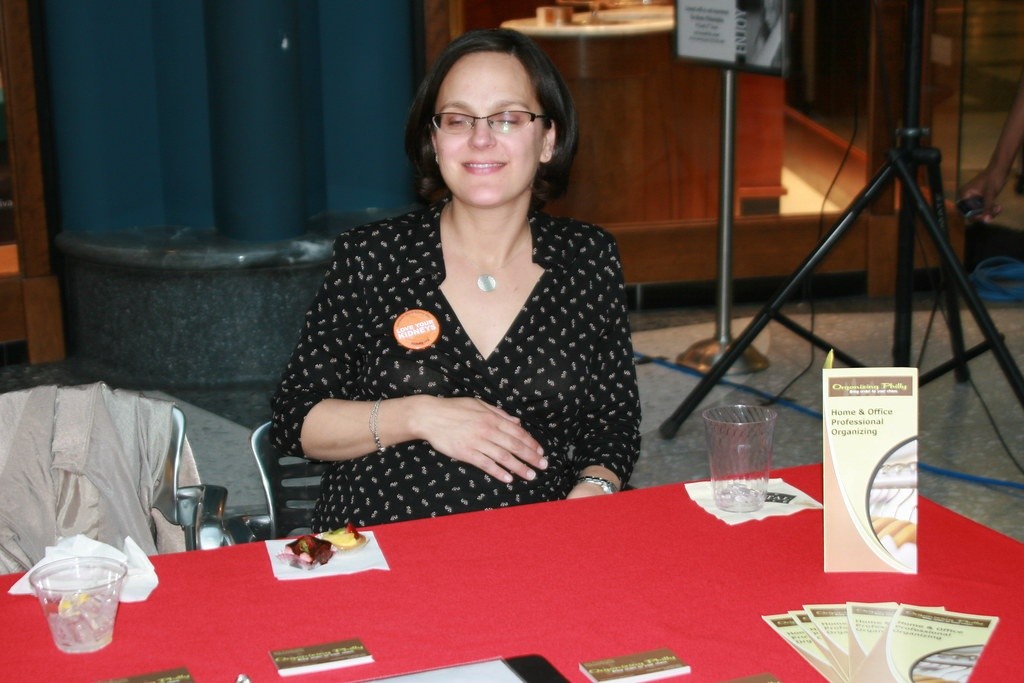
[657,1,1023,440]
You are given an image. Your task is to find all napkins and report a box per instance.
[6,535,159,602]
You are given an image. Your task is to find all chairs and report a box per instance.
[0,380,316,574]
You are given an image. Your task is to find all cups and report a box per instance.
[703,405,778,513]
[29,556,128,654]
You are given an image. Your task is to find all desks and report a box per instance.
[498,8,788,230]
[0,463,1024,683]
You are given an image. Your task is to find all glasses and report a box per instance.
[433,109,551,134]
